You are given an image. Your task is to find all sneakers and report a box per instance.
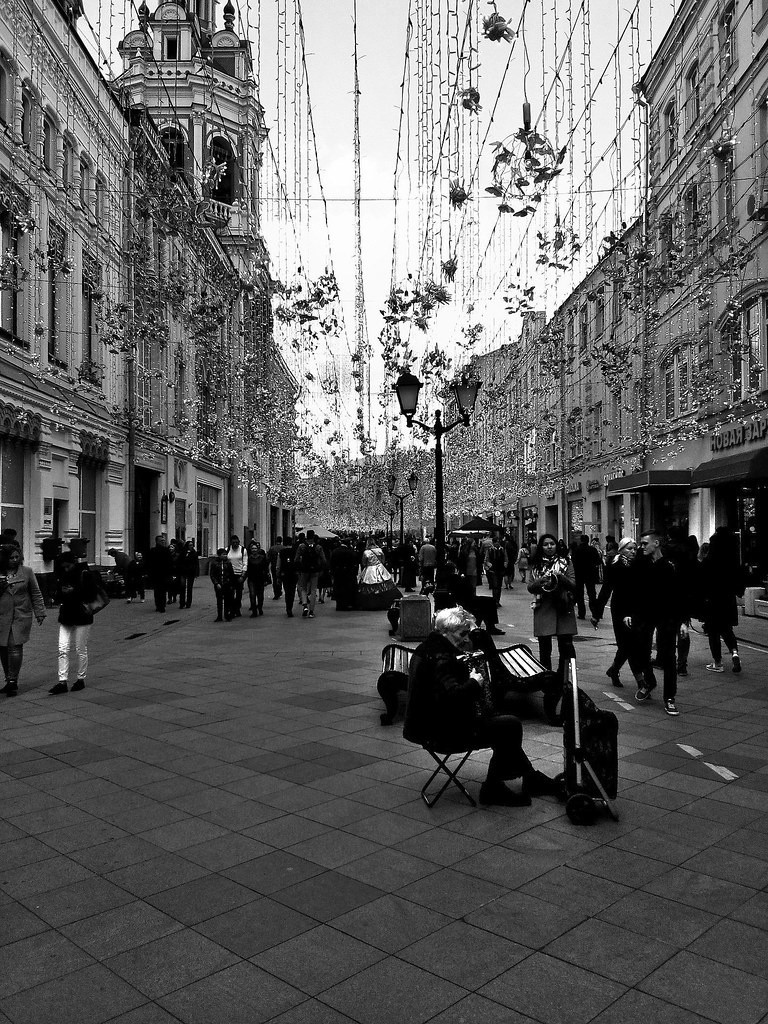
[676,666,687,677]
[649,658,663,670]
[663,695,679,715]
[302,605,308,617]
[71,679,85,691]
[541,696,558,724]
[704,662,724,674]
[307,612,316,617]
[605,667,624,688]
[522,772,555,797]
[1,683,18,697]
[48,682,68,693]
[635,682,657,701]
[478,781,532,807]
[731,649,742,673]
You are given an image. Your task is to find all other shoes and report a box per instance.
[497,603,502,609]
[404,588,415,592]
[576,614,585,619]
[225,616,231,622]
[153,607,166,612]
[212,617,223,623]
[141,599,145,603]
[484,625,505,635]
[167,599,177,604]
[318,598,326,604]
[178,603,192,609]
[248,610,264,619]
[125,596,133,603]
[504,586,513,590]
[272,592,282,600]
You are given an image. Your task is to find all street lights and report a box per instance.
[390,372,484,611]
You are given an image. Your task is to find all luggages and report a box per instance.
[554,657,618,826]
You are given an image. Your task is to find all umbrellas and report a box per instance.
[295,525,338,538]
[454,518,502,533]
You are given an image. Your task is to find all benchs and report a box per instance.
[377,642,417,731]
[468,618,565,728]
[100,571,123,596]
[386,579,435,637]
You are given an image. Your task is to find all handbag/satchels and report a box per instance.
[74,569,110,618]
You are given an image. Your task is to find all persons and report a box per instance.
[0,529,768,715]
[404,606,559,809]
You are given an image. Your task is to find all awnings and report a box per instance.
[691,447,768,488]
[608,470,693,491]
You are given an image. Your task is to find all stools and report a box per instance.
[418,741,480,809]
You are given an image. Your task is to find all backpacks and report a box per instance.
[303,541,323,573]
